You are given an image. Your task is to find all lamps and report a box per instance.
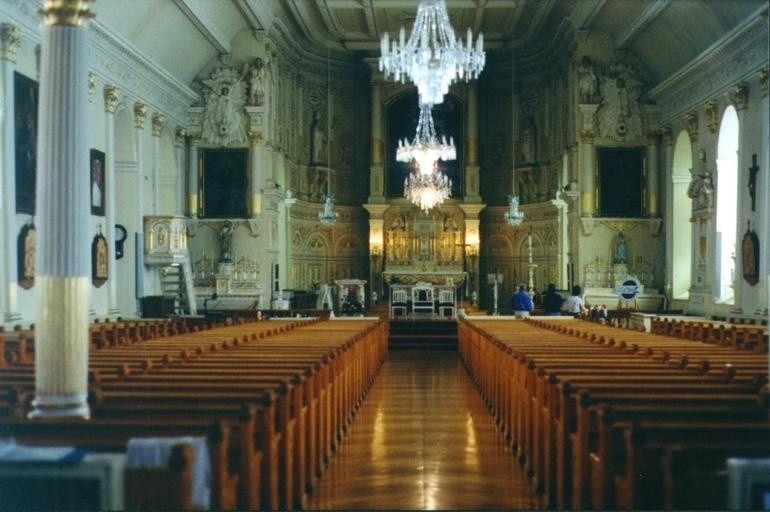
[319,46,338,226]
[378,0,486,211]
[504,8,525,226]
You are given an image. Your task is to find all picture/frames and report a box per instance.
[91,148,105,216]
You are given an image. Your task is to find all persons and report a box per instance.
[612,228,628,263]
[579,54,600,105]
[440,215,463,263]
[542,281,563,316]
[589,304,599,320]
[312,280,334,319]
[518,115,539,166]
[565,284,595,319]
[329,278,340,314]
[16,83,36,211]
[509,283,535,319]
[211,81,241,126]
[91,159,103,208]
[615,77,638,125]
[309,107,328,165]
[215,219,236,263]
[599,304,607,320]
[385,217,409,261]
[246,57,266,106]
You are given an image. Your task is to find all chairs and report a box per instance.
[389,280,456,321]
